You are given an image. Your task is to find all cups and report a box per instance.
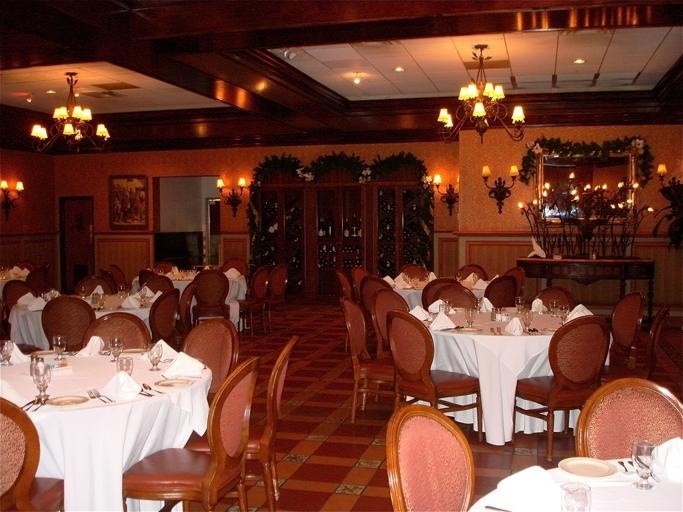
[0,339,13,366]
[515,297,524,314]
[116,357,133,379]
[559,305,569,326]
[111,335,122,363]
[442,298,452,312]
[561,484,592,512]
[465,307,476,329]
[438,304,448,315]
[32,362,51,405]
[549,300,558,316]
[631,441,657,490]
[146,344,163,371]
[521,311,533,330]
[52,336,65,361]
[472,297,484,315]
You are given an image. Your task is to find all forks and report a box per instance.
[93,389,112,403]
[496,326,502,336]
[490,327,497,337]
[160,374,202,380]
[86,389,108,406]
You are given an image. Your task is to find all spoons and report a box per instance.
[532,327,542,335]
[142,383,163,394]
[527,328,537,336]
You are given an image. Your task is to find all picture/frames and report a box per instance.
[108,174,148,230]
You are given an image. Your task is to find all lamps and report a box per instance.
[656,164,665,189]
[482,165,496,189]
[216,178,230,200]
[0,180,9,205]
[236,177,246,198]
[9,181,24,204]
[31,72,111,152]
[505,165,519,189]
[434,173,448,195]
[437,45,525,144]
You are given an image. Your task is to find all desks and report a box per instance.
[406,310,613,446]
[516,257,655,331]
[0,351,212,512]
[84,312,151,349]
[132,274,247,333]
[8,294,154,351]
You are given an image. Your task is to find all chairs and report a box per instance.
[432,285,478,310]
[143,275,174,294]
[455,264,488,281]
[3,280,31,317]
[24,265,48,297]
[139,269,158,290]
[122,355,260,512]
[0,397,64,512]
[422,278,462,312]
[222,257,249,278]
[537,286,575,313]
[181,318,239,406]
[176,276,200,336]
[343,299,396,424]
[399,264,427,281]
[485,275,517,310]
[511,315,610,462]
[386,405,475,512]
[192,270,230,329]
[185,335,299,512]
[98,268,123,293]
[601,303,670,382]
[504,267,525,305]
[262,263,289,336]
[110,264,132,288]
[375,289,410,346]
[352,266,375,337]
[237,266,269,336]
[360,276,393,403]
[575,378,683,462]
[42,296,96,352]
[75,275,112,296]
[149,288,180,350]
[335,271,374,353]
[610,291,646,365]
[386,310,483,443]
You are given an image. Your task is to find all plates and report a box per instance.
[558,457,617,478]
[44,395,88,406]
[154,379,195,387]
